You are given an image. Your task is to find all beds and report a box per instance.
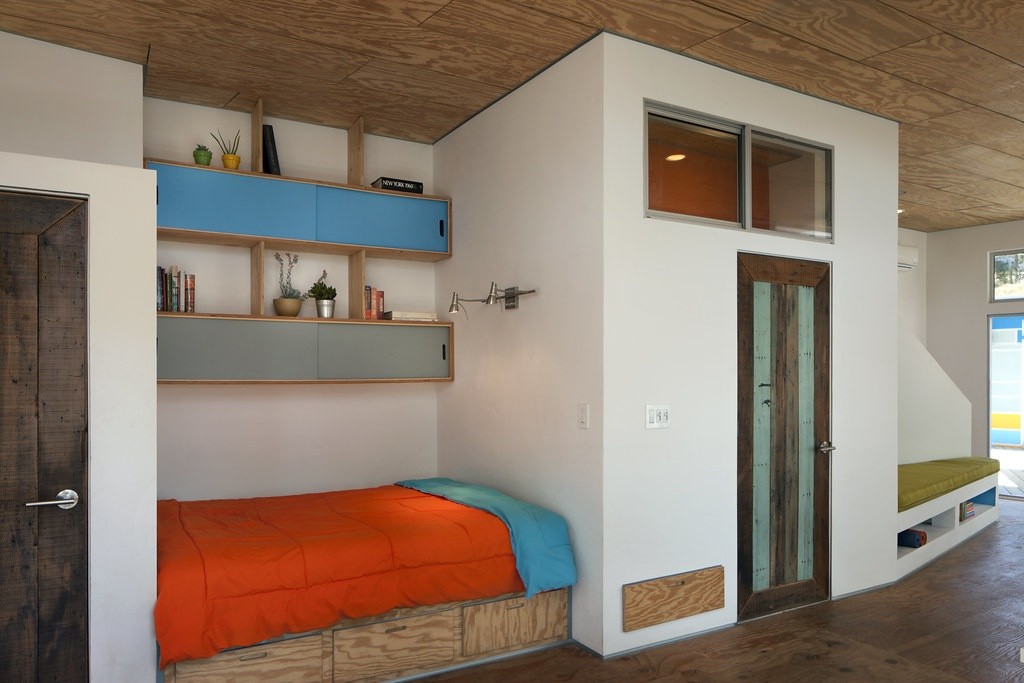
[153,477,577,683]
[897,458,1000,576]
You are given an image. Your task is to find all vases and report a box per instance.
[274,252,328,316]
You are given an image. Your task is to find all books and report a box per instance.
[365,286,439,321]
[960,501,976,523]
[157,264,196,312]
[371,176,424,194]
[263,124,282,175]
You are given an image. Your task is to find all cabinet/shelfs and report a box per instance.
[143,155,452,264]
[156,311,455,384]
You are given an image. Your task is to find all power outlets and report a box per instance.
[645,404,673,428]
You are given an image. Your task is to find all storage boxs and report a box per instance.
[960,500,975,522]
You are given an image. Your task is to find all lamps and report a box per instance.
[487,282,536,304]
[448,292,505,313]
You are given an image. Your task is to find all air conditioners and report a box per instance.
[899,244,919,271]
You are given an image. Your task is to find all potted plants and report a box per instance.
[308,283,338,318]
[193,143,213,165]
[210,129,241,171]
